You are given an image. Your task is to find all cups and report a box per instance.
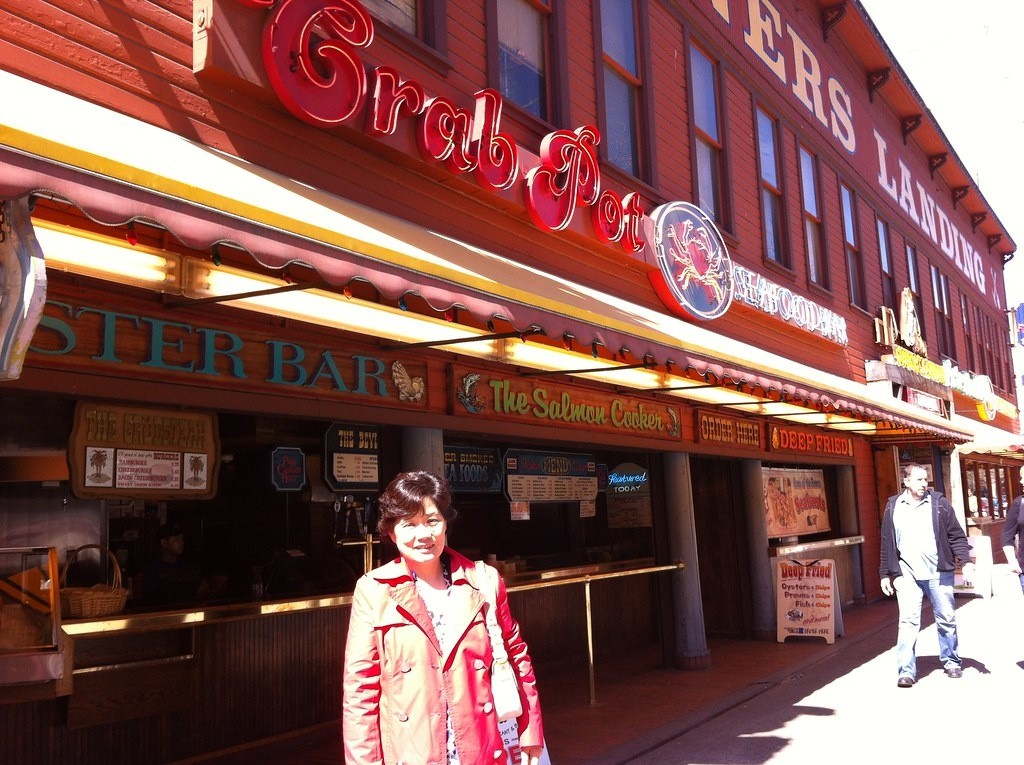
[488,554,527,575]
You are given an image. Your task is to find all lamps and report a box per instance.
[30,217,878,436]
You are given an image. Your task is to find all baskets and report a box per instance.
[60,545,130,618]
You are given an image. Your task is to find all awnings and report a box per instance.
[0,143,974,444]
[958,444,1024,465]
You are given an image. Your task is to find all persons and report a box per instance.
[968,488,977,512]
[343,471,544,765]
[880,463,976,687]
[129,524,207,610]
[1002,465,1024,594]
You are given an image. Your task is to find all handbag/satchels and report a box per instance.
[475,560,523,722]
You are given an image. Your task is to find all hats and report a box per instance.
[155,521,186,538]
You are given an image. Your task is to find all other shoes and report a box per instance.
[898,672,917,686]
[944,667,962,677]
